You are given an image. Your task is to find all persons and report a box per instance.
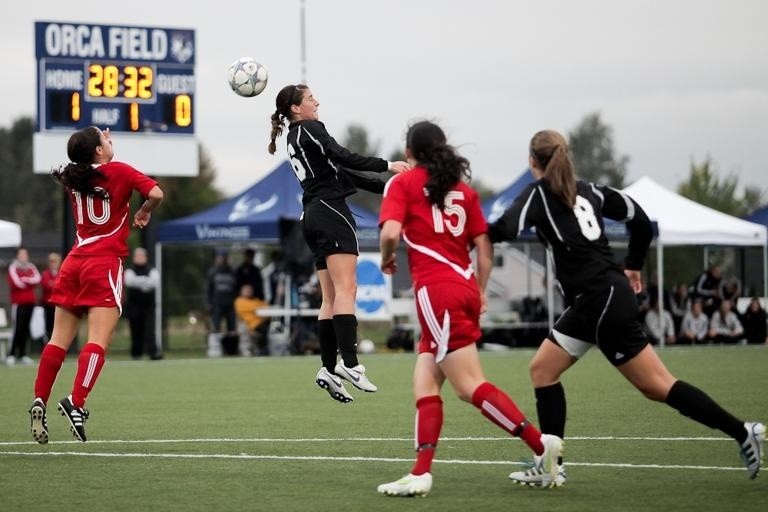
[706,299,747,344]
[200,247,238,332]
[266,83,413,404]
[123,246,166,361]
[643,303,677,347]
[261,252,281,303]
[40,252,64,347]
[679,298,710,341]
[463,128,765,488]
[232,284,273,356]
[235,248,265,302]
[27,125,166,445]
[375,120,567,498]
[691,265,723,301]
[668,281,693,316]
[735,295,768,344]
[635,292,650,333]
[648,269,671,309]
[3,246,43,366]
[722,276,743,303]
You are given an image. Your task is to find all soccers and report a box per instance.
[229,57,268,97]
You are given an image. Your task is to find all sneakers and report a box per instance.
[335,359,377,391]
[29,397,48,444]
[740,423,766,480]
[509,434,567,488]
[57,395,89,442]
[377,472,433,497]
[315,367,353,402]
